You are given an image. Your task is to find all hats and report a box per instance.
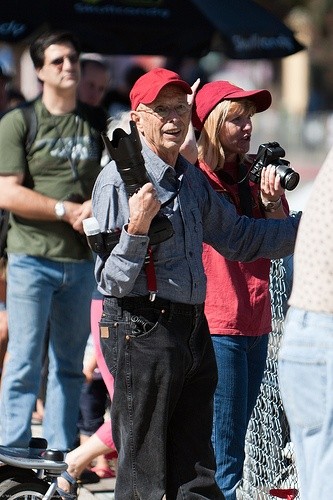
[190,80,273,129]
[129,68,193,110]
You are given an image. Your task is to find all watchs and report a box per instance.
[55,201,65,220]
[260,193,282,208]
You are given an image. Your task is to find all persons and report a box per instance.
[191,81,290,500]
[0,31,105,484]
[0,53,116,478]
[55,78,202,500]
[91,69,302,500]
[278,147,333,500]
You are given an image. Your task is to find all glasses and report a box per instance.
[46,54,79,67]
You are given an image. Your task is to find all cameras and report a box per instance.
[249,141,300,191]
[81,121,176,254]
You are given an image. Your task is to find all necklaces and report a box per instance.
[51,115,79,182]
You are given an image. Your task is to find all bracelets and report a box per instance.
[262,203,283,214]
